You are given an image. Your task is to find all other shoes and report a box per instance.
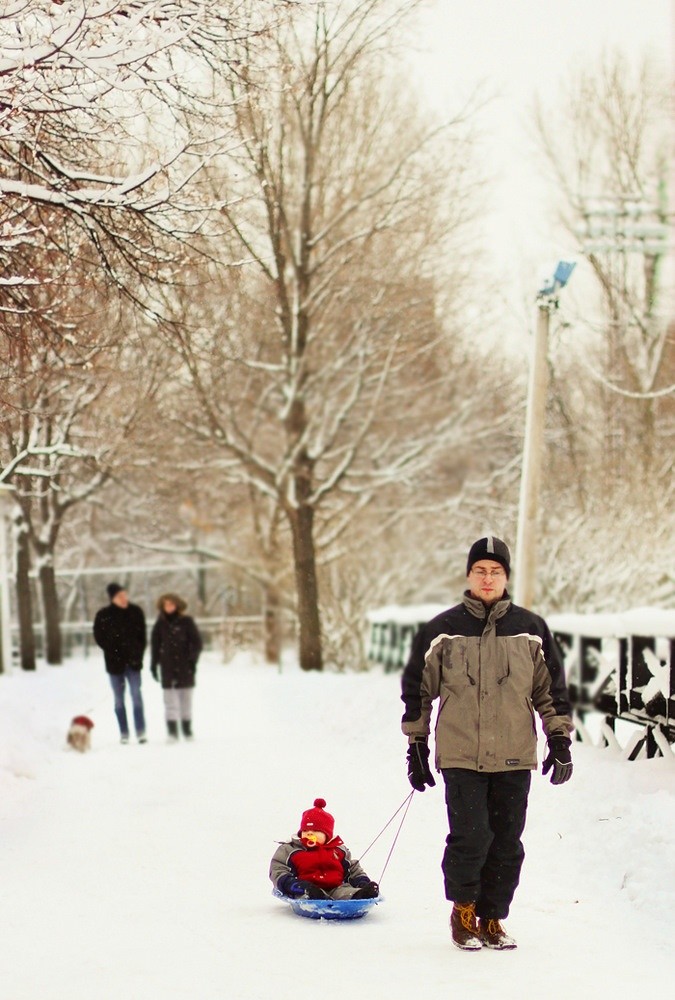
[138,734,146,742]
[119,734,129,743]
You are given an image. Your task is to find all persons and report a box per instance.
[266,796,381,899]
[91,581,149,746]
[148,591,203,745]
[402,534,575,951]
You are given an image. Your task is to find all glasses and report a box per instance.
[470,568,505,576]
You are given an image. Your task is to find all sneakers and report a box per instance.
[474,915,516,951]
[452,901,483,950]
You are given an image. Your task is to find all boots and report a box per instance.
[182,719,192,735]
[167,721,179,737]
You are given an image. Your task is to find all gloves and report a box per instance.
[152,666,158,680]
[284,876,308,894]
[543,735,573,783]
[405,741,437,792]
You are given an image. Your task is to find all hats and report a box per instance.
[107,583,123,600]
[295,799,333,841]
[468,537,510,579]
